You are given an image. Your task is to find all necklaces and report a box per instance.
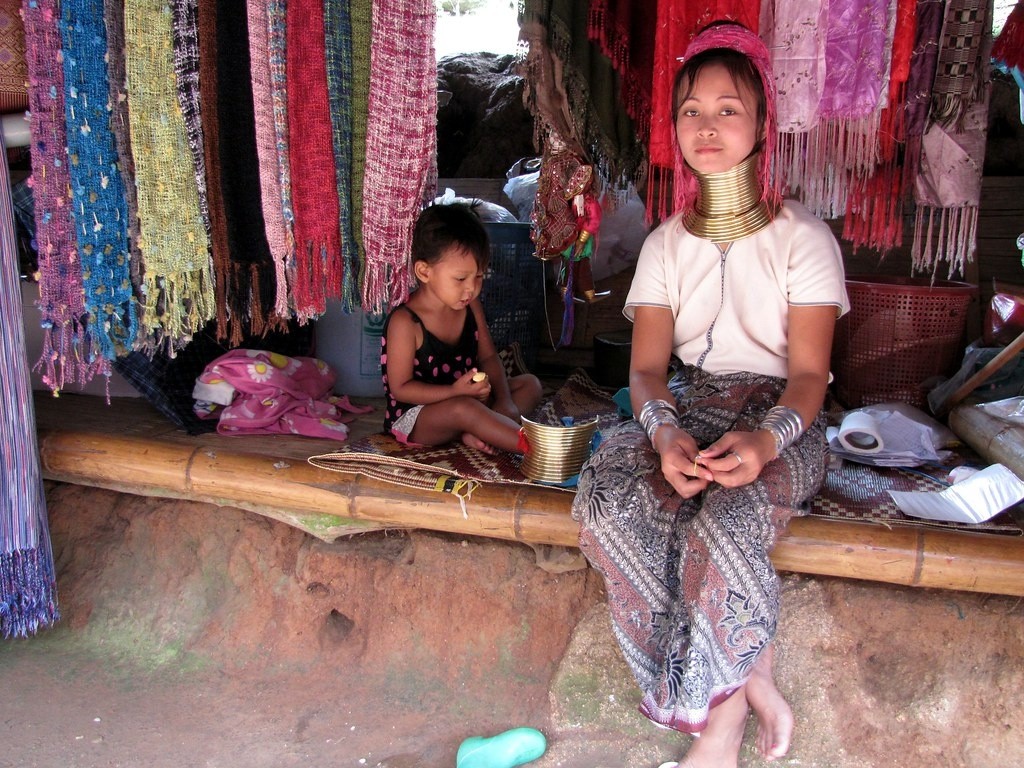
[681,153,782,244]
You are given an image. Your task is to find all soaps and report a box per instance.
[457,727,547,768]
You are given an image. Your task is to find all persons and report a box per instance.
[378,205,542,456]
[569,45,851,767]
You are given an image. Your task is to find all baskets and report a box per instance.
[476,221,540,349]
[842,276,977,410]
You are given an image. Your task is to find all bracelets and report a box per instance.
[757,405,804,461]
[638,398,679,452]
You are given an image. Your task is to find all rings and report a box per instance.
[731,452,743,464]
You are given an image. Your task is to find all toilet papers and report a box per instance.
[837,411,884,453]
